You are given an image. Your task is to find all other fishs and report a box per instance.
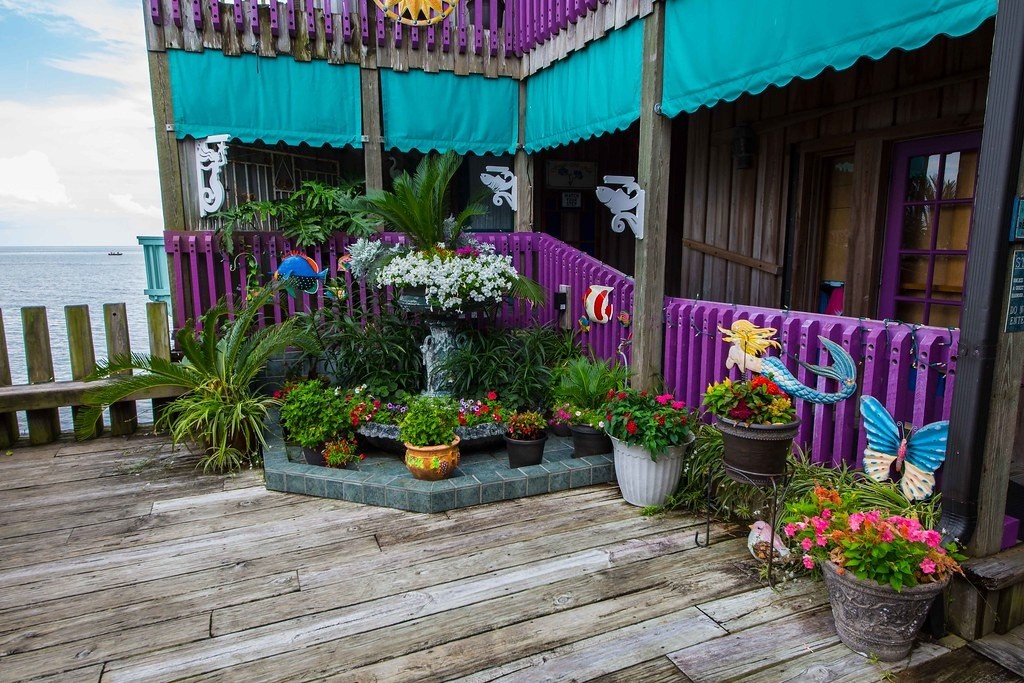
[336,254,353,272]
[577,316,591,334]
[618,310,633,328]
[595,182,642,215]
[268,249,328,294]
[244,287,276,303]
[324,288,349,299]
[479,170,514,193]
[581,285,615,325]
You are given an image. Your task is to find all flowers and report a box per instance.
[591,388,701,463]
[504,408,548,440]
[550,401,607,432]
[333,384,507,426]
[321,435,366,469]
[781,477,968,593]
[276,382,297,400]
[343,217,519,319]
[700,377,797,429]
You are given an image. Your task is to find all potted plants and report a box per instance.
[73,277,321,479]
[280,378,350,464]
[396,394,461,480]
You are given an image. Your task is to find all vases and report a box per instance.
[603,427,696,507]
[713,412,800,487]
[357,421,509,454]
[329,462,347,469]
[818,558,952,663]
[397,285,494,401]
[502,433,546,469]
[566,421,613,459]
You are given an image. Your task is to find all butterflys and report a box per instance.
[858,395,950,503]
